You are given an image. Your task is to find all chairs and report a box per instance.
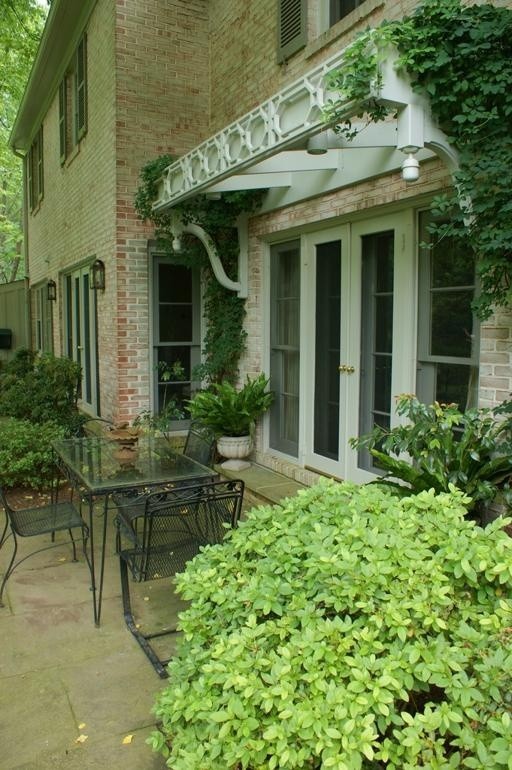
[119,477,245,678]
[2,476,100,626]
[114,420,219,557]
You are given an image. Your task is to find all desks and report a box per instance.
[48,433,218,628]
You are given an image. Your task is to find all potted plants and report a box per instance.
[182,372,277,471]
